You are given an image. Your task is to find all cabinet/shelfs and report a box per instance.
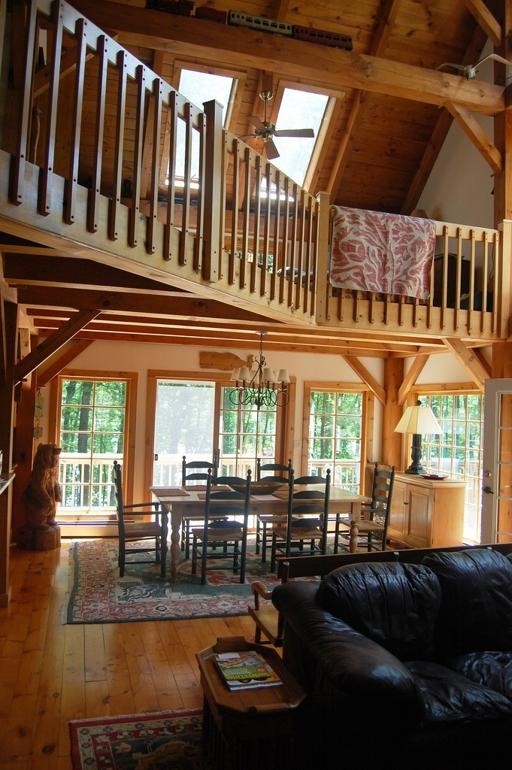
[386,475,465,547]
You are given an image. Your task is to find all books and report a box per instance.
[214,650,285,692]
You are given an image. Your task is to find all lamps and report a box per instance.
[393,405,443,474]
[229,332,291,407]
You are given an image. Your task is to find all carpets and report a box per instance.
[68,541,319,624]
[68,707,212,770]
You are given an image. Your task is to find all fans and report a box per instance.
[239,71,315,160]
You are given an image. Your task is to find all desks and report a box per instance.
[198,635,309,770]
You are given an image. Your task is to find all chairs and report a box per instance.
[114,456,396,585]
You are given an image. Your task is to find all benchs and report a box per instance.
[247,545,512,649]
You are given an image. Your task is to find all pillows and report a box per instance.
[424,551,512,650]
[321,562,442,660]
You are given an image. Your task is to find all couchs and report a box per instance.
[273,550,512,770]
